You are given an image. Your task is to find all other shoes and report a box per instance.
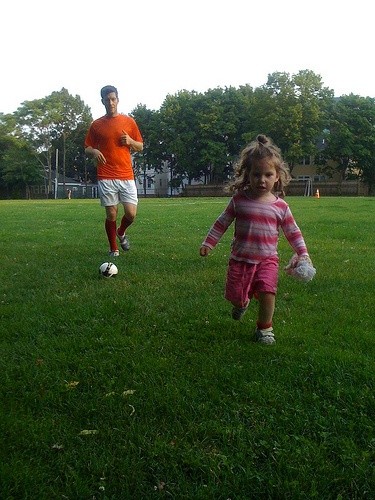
[116,232,131,251]
[254,327,277,346]
[108,249,121,257]
[231,298,250,321]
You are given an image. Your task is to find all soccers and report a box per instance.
[99,262,119,280]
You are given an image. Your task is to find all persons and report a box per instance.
[200,136,316,347]
[85,85,143,258]
[67,189,71,199]
